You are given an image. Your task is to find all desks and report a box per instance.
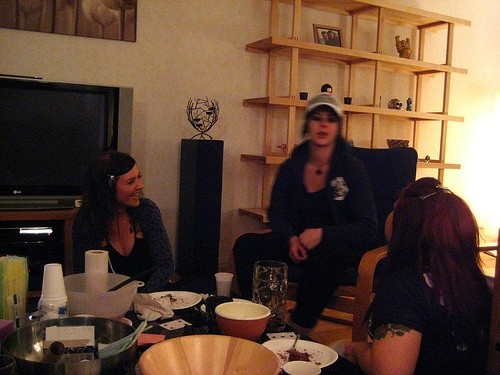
[175,139,224,295]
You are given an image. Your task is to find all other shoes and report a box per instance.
[285,319,312,338]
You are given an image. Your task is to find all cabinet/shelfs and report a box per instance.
[239,0,471,223]
[0,206,80,296]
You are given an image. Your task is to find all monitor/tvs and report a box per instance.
[0,78,120,212]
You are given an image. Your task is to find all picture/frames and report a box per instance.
[313,24,342,47]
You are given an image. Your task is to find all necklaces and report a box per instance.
[312,157,329,175]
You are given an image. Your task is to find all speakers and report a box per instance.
[177,139,224,293]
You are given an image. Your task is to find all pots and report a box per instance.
[2,317,137,375]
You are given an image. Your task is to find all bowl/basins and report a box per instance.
[214,302,271,341]
[138,335,280,375]
[63,272,137,319]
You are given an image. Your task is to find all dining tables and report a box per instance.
[126,294,363,375]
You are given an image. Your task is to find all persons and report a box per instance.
[72,152,170,294]
[234,93,377,333]
[322,30,341,46]
[338,177,489,375]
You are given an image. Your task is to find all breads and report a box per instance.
[288,349,311,364]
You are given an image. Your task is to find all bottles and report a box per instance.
[406,98,412,111]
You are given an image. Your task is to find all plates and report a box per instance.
[147,290,202,310]
[200,298,252,312]
[262,339,338,368]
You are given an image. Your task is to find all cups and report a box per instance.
[40,263,69,319]
[205,296,233,334]
[252,260,287,333]
[299,92,309,100]
[282,361,321,374]
[344,97,353,105]
[215,273,234,297]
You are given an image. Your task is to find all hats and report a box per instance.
[305,93,345,117]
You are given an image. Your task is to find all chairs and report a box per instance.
[287,147,418,346]
